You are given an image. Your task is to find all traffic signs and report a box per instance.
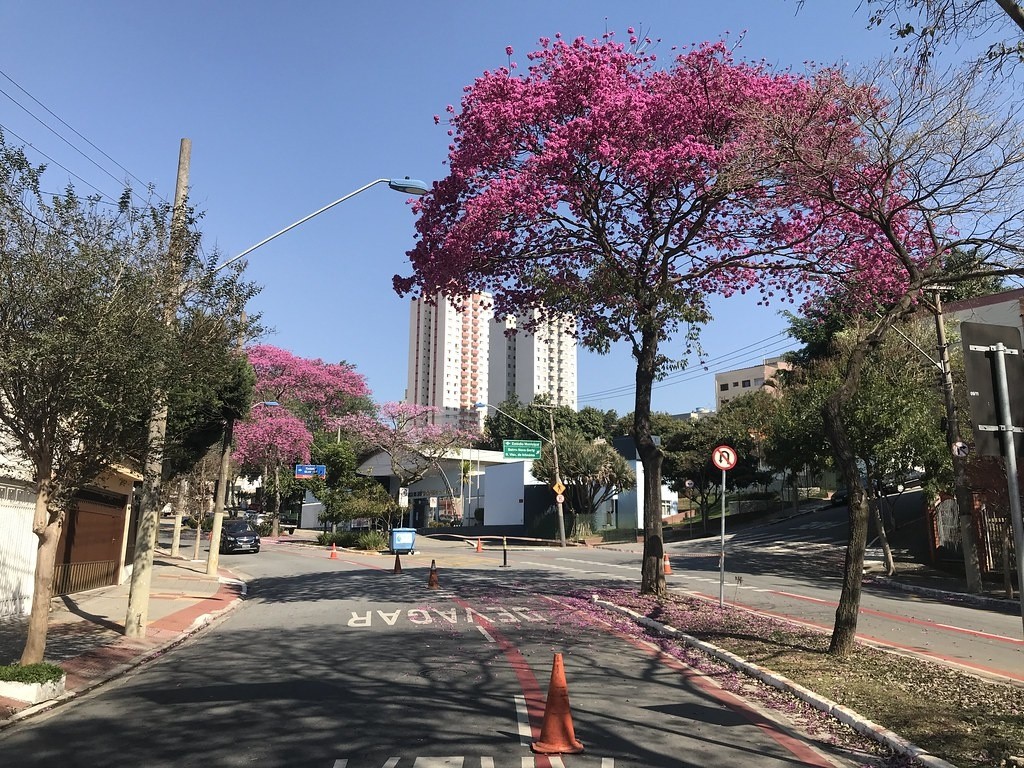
[503,440,541,448]
[503,448,541,458]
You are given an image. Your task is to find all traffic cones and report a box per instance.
[664,554,673,575]
[394,553,403,574]
[425,559,442,589]
[531,653,584,753]
[328,543,338,559]
[476,538,484,553]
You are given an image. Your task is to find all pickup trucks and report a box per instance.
[261,514,299,535]
[181,512,215,527]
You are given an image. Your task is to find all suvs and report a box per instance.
[247,514,270,529]
[208,521,261,554]
[243,510,257,523]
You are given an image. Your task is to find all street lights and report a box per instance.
[122,138,430,635]
[474,404,566,547]
[205,402,280,576]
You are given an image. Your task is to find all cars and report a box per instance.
[830,478,884,506]
[160,505,172,516]
[880,469,928,493]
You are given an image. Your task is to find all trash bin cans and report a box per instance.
[388,527,416,555]
